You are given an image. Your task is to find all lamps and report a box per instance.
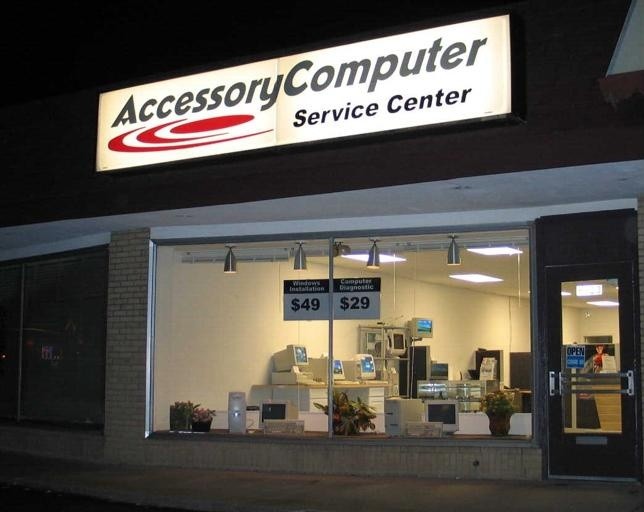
[222,234,461,274]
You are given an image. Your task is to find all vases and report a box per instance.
[191,421,210,432]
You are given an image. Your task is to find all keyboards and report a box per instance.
[407,422,443,439]
[263,419,304,434]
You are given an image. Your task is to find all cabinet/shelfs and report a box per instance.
[269,382,388,413]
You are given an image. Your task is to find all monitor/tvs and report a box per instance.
[272,317,433,384]
[417,380,449,402]
[259,399,298,428]
[424,400,459,437]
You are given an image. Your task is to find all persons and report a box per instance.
[592,346,605,375]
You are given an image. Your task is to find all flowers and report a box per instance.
[190,404,216,423]
[312,388,377,436]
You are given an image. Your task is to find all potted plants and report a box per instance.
[482,389,514,435]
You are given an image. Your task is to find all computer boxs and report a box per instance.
[229,391,246,433]
[384,399,422,434]
